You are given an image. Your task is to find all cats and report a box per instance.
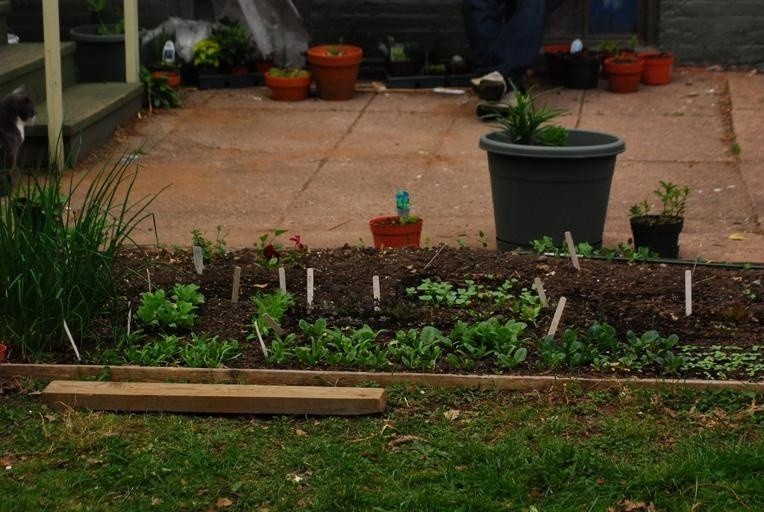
[0,83,38,197]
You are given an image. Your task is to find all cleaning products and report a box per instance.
[160,39,177,65]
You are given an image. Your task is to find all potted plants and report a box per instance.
[640,51,678,89]
[560,53,605,90]
[477,80,627,253]
[70,0,148,83]
[630,181,689,262]
[306,44,364,101]
[600,38,643,93]
[10,184,68,236]
[368,191,423,248]
[266,64,313,101]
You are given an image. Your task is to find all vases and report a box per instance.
[154,70,184,92]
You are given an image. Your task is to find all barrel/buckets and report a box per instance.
[478,128,626,256]
